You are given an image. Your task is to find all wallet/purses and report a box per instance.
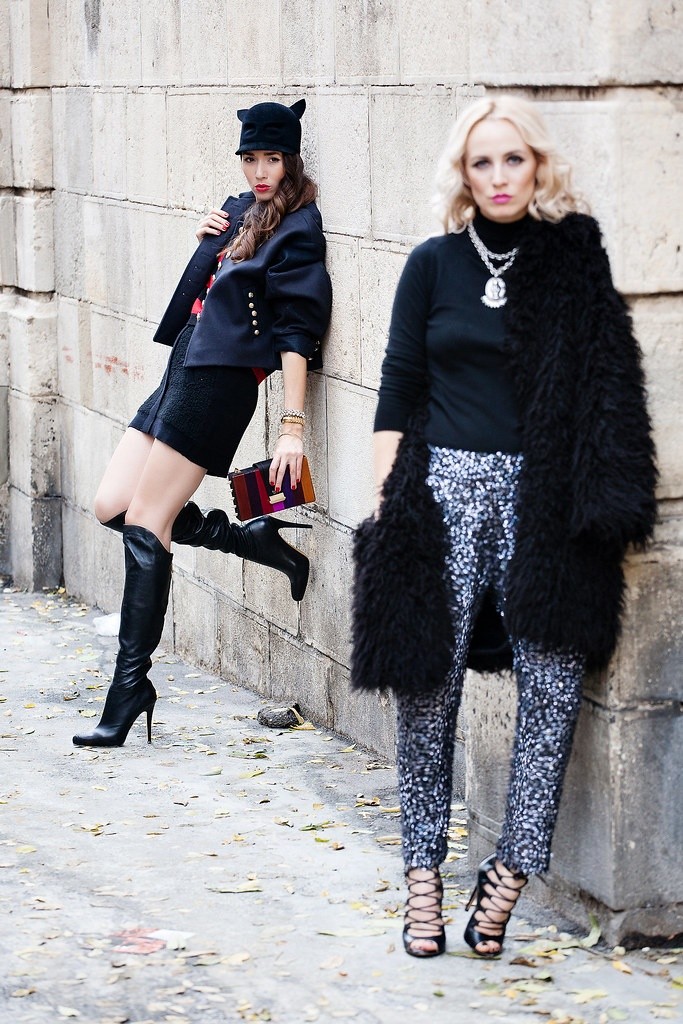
[229,455,316,521]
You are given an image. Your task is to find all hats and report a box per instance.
[235,97,306,153]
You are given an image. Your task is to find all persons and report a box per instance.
[71,98,333,748]
[351,94,662,959]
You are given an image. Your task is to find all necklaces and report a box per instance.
[467,219,520,309]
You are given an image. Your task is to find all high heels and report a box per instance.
[403,863,447,959]
[464,848,528,959]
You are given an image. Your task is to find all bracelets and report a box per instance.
[280,408,306,426]
[278,434,302,442]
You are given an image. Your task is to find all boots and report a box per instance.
[101,497,313,602]
[72,525,179,746]
